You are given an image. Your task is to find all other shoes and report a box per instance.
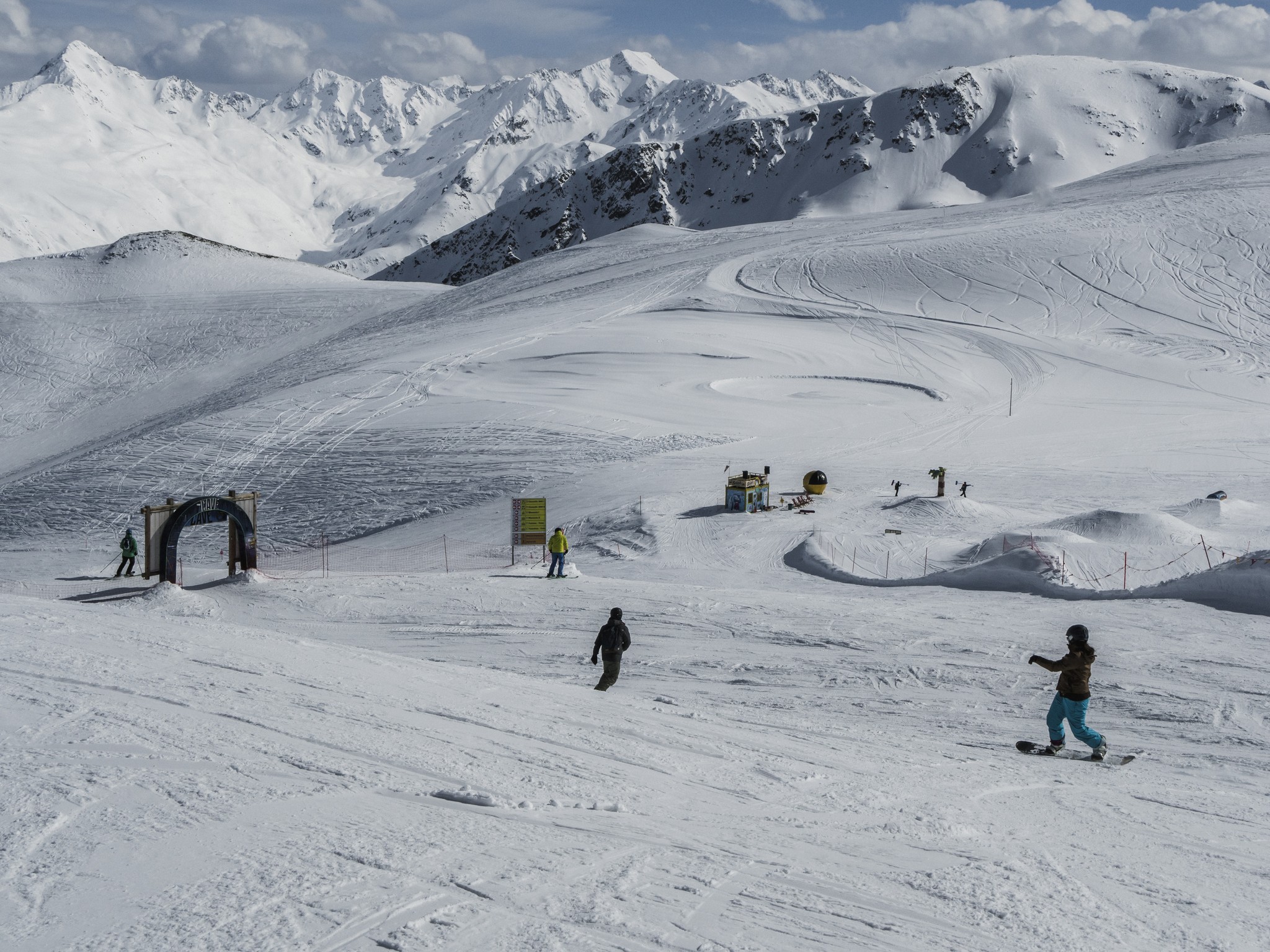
[1091,736,1107,760]
[117,573,123,576]
[126,573,134,576]
[556,575,564,578]
[1045,743,1066,754]
[548,573,555,577]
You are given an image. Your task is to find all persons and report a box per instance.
[116,529,138,577]
[959,482,971,497]
[895,481,902,496]
[546,528,568,578]
[591,607,631,692]
[1028,624,1108,762]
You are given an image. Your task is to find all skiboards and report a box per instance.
[105,571,143,581]
[538,575,579,580]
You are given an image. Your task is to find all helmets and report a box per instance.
[126,529,132,535]
[611,607,623,620]
[1066,625,1089,643]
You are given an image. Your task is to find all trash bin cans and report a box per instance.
[788,504,793,510]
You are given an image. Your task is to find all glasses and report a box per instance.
[1066,635,1073,642]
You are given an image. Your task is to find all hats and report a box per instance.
[555,528,562,533]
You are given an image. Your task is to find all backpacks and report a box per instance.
[602,623,625,651]
[123,538,133,553]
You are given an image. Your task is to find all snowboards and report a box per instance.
[794,509,815,515]
[1015,740,1135,766]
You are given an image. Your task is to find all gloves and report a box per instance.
[134,552,138,555]
[591,654,598,665]
[549,549,552,553]
[564,549,568,555]
[1029,658,1032,665]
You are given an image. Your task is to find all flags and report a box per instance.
[724,466,729,472]
